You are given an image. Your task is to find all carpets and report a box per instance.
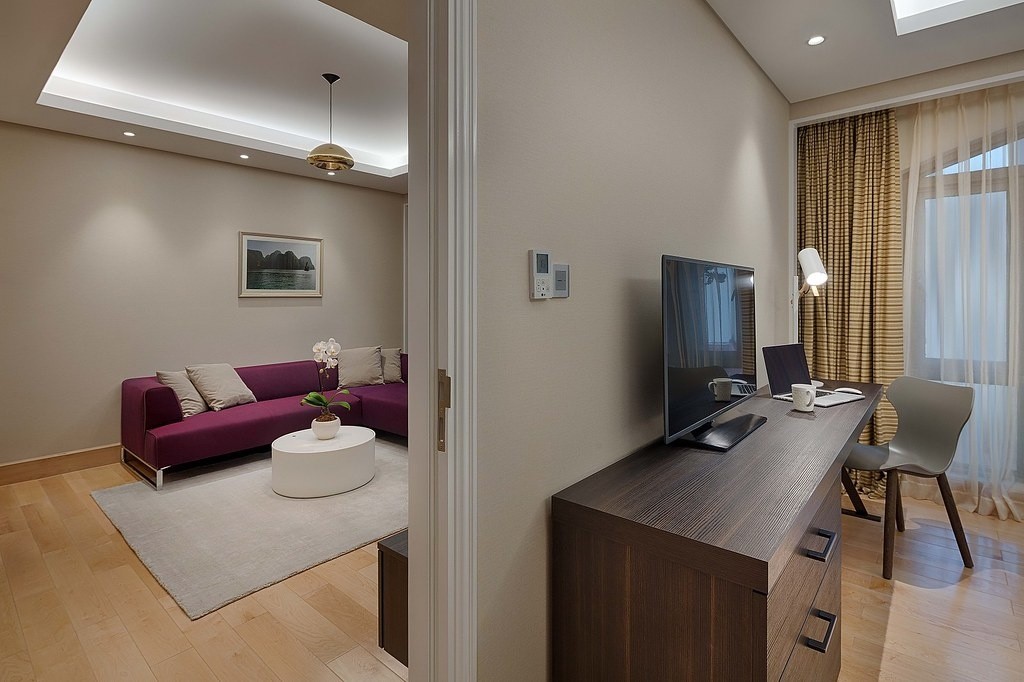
[89,437,409,620]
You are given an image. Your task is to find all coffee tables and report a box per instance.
[271,426,376,499]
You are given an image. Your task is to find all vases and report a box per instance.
[311,416,341,439]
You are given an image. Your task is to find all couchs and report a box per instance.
[120,353,408,490]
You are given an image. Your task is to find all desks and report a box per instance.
[552,377,883,682]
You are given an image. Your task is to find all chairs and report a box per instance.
[844,375,976,579]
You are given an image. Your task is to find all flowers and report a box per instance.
[298,337,351,421]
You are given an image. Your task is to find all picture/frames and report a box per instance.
[237,231,325,298]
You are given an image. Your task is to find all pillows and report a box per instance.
[184,363,258,412]
[155,370,208,418]
[380,348,404,383]
[337,345,385,390]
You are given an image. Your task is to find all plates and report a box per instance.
[810,380,824,388]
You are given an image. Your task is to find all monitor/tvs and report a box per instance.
[661,254,768,450]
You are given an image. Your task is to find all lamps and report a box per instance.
[306,74,354,171]
[793,248,828,343]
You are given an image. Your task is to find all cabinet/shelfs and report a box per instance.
[377,529,408,669]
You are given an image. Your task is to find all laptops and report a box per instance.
[731,384,756,396]
[763,344,865,407]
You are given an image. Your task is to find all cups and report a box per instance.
[707,377,733,401]
[791,383,817,412]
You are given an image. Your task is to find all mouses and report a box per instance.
[834,387,862,395]
